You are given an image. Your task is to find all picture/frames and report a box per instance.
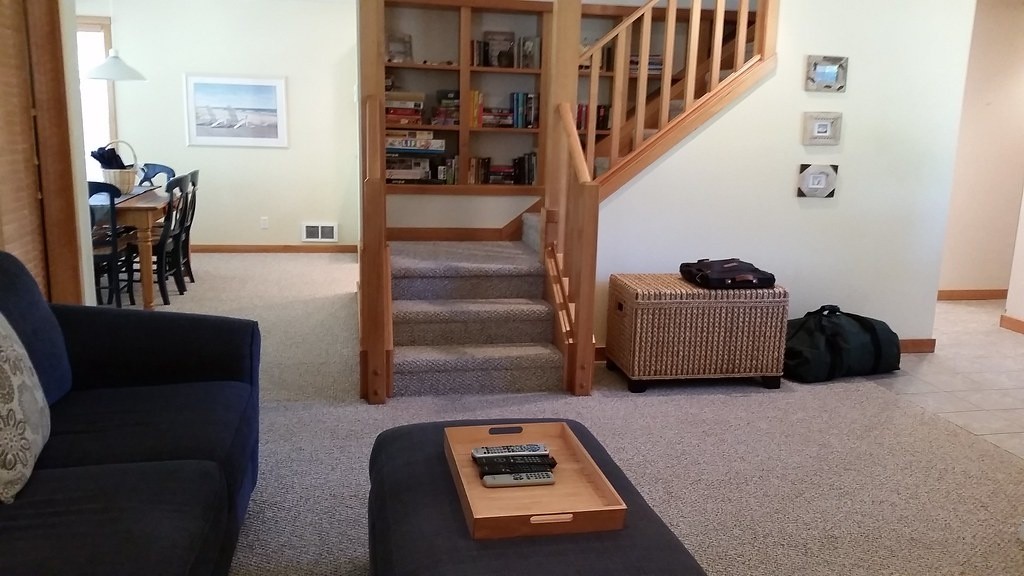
[804,111,843,146]
[185,76,290,147]
[805,55,848,94]
[797,164,839,199]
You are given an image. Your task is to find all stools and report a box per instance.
[607,272,791,393]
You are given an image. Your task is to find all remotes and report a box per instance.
[471,444,556,488]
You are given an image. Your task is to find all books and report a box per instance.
[386,30,663,185]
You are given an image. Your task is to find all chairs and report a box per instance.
[87,181,136,308]
[108,164,202,307]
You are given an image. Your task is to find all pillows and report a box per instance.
[0,248,75,402]
[0,312,50,506]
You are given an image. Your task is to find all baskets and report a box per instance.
[102,169,136,194]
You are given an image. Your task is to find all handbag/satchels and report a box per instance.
[679,259,776,290]
[784,304,901,383]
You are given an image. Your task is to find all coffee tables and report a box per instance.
[368,418,708,576]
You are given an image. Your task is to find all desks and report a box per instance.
[90,185,198,310]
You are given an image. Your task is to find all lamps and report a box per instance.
[80,49,146,82]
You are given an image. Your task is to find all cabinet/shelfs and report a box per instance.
[385,0,757,197]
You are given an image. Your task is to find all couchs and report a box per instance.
[0,303,261,576]
